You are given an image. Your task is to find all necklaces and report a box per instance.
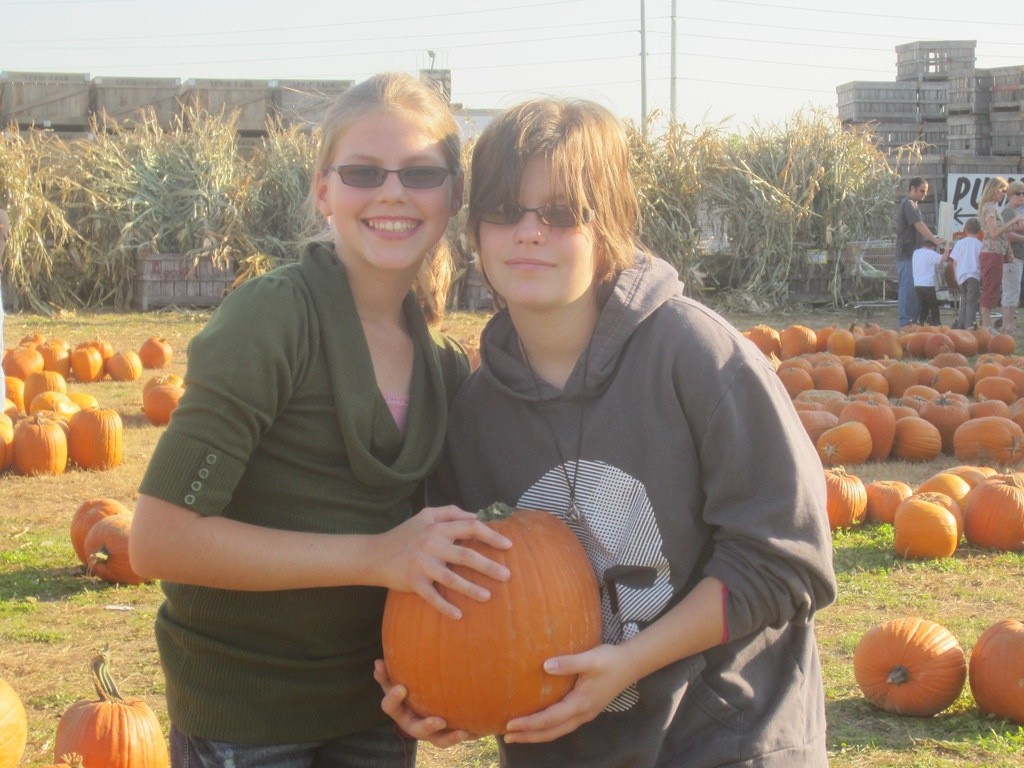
[511,317,602,527]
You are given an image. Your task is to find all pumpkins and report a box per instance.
[740,320,1024,725]
[0,332,188,768]
[381,500,603,736]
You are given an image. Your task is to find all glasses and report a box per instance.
[327,161,455,191]
[475,197,598,229]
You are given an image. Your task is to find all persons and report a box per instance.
[912,237,951,328]
[896,176,944,326]
[126,69,474,768]
[427,94,838,768]
[950,219,983,331]
[1001,181,1024,333]
[979,175,1024,334]
